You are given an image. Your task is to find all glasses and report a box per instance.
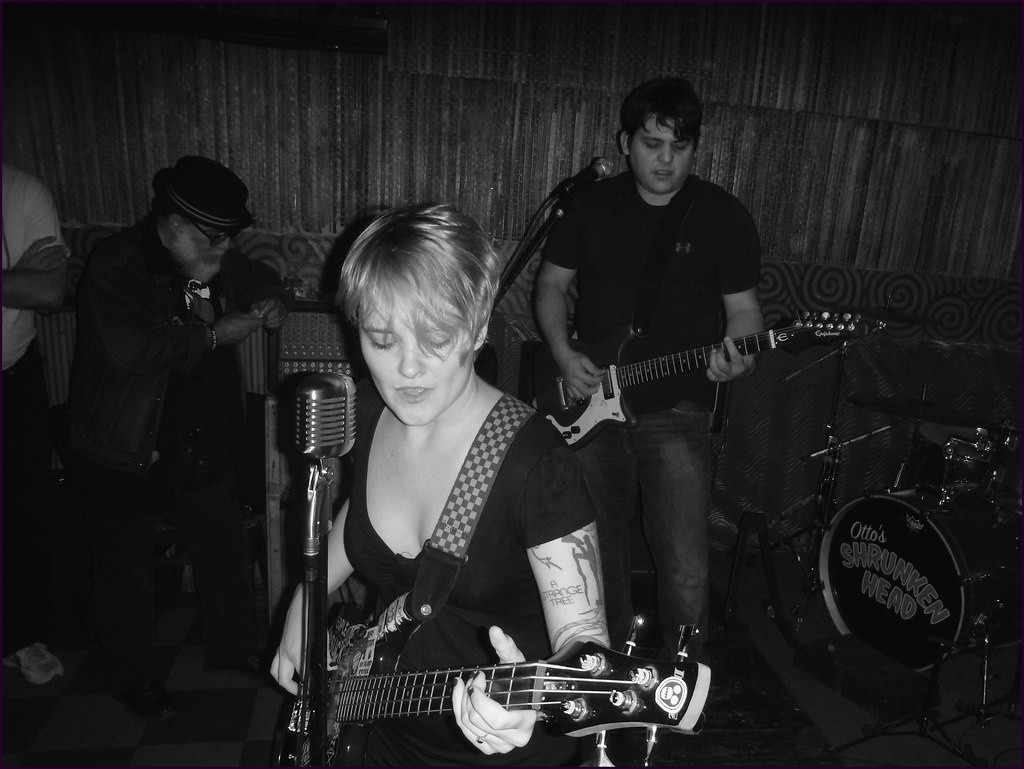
[188,219,240,247]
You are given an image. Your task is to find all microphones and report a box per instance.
[562,158,613,194]
[853,314,887,328]
[279,371,356,461]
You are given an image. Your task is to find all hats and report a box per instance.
[152,156,254,231]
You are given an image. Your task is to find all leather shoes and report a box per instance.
[113,681,180,723]
[204,653,266,680]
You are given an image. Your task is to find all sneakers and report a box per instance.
[3,641,63,685]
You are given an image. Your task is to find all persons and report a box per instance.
[530,76,768,736]
[0,159,72,685]
[75,157,302,720]
[268,202,613,769]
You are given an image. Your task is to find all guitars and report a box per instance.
[264,578,715,768]
[522,306,888,450]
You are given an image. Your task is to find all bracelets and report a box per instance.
[206,324,217,351]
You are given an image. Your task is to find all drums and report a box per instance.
[817,492,991,673]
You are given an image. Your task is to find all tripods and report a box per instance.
[769,327,1022,768]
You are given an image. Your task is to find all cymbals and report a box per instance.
[847,395,1001,431]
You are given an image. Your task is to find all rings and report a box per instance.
[279,313,284,321]
